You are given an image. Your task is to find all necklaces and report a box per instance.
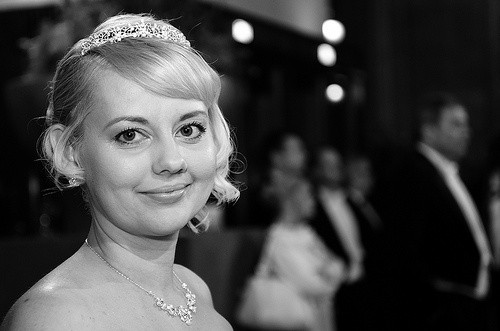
[86,238,196,325]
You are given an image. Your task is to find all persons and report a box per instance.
[234,91,500,331]
[1,13,240,331]
